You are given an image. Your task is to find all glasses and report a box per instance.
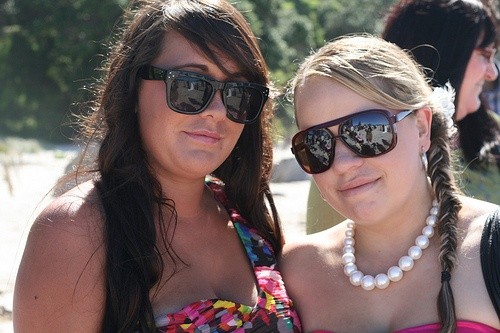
[474,46,496,62]
[290,109,414,174]
[136,62,270,123]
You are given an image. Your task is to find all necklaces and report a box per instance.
[340,197,445,291]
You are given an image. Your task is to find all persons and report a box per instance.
[237,86,250,121]
[305,0,500,236]
[170,78,180,106]
[202,82,214,102]
[277,34,500,333]
[304,131,332,165]
[12,0,303,332]
[345,119,393,155]
